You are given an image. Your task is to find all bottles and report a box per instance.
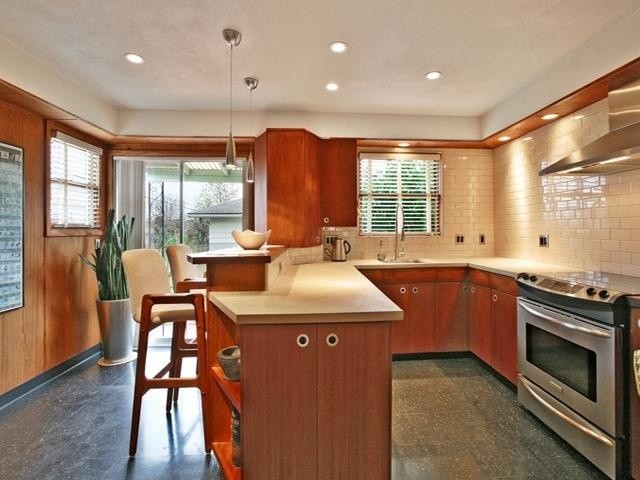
[231,406,243,469]
[376,240,387,261]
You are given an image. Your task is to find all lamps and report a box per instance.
[244,74,259,183]
[218,28,243,168]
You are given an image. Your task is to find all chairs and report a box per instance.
[116,248,215,461]
[164,241,207,402]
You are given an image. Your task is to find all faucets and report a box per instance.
[393,203,405,259]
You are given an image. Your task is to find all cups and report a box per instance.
[216,343,243,382]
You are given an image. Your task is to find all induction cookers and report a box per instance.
[514,270,640,297]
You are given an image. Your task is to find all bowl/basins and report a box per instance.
[232,227,272,250]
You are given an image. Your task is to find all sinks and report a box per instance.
[393,258,423,263]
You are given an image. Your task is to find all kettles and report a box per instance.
[330,237,353,263]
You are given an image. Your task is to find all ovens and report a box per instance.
[508,295,630,480]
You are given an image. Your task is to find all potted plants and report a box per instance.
[77,209,139,365]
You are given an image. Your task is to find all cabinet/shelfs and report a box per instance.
[357,268,384,292]
[466,265,519,389]
[383,267,434,358]
[624,307,640,478]
[202,297,392,480]
[434,267,466,354]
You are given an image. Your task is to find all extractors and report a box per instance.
[538,122,640,175]
